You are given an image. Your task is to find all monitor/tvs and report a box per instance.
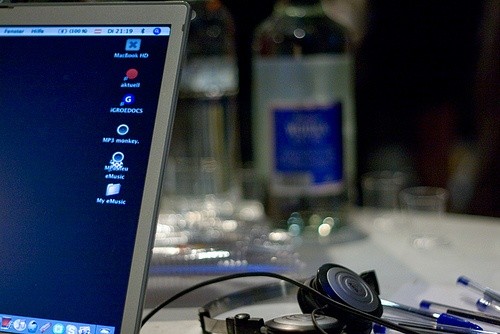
[0,0,194,333]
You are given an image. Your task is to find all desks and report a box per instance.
[141,207,500,334]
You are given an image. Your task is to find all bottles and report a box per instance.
[165,0,244,215]
[246,1,359,234]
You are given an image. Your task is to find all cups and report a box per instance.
[399,187,448,252]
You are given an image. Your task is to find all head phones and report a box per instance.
[197,262,384,334]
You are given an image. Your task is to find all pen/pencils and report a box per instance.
[477,297,500,315]
[457,275,500,302]
[378,315,499,334]
[420,298,499,327]
[380,298,483,331]
